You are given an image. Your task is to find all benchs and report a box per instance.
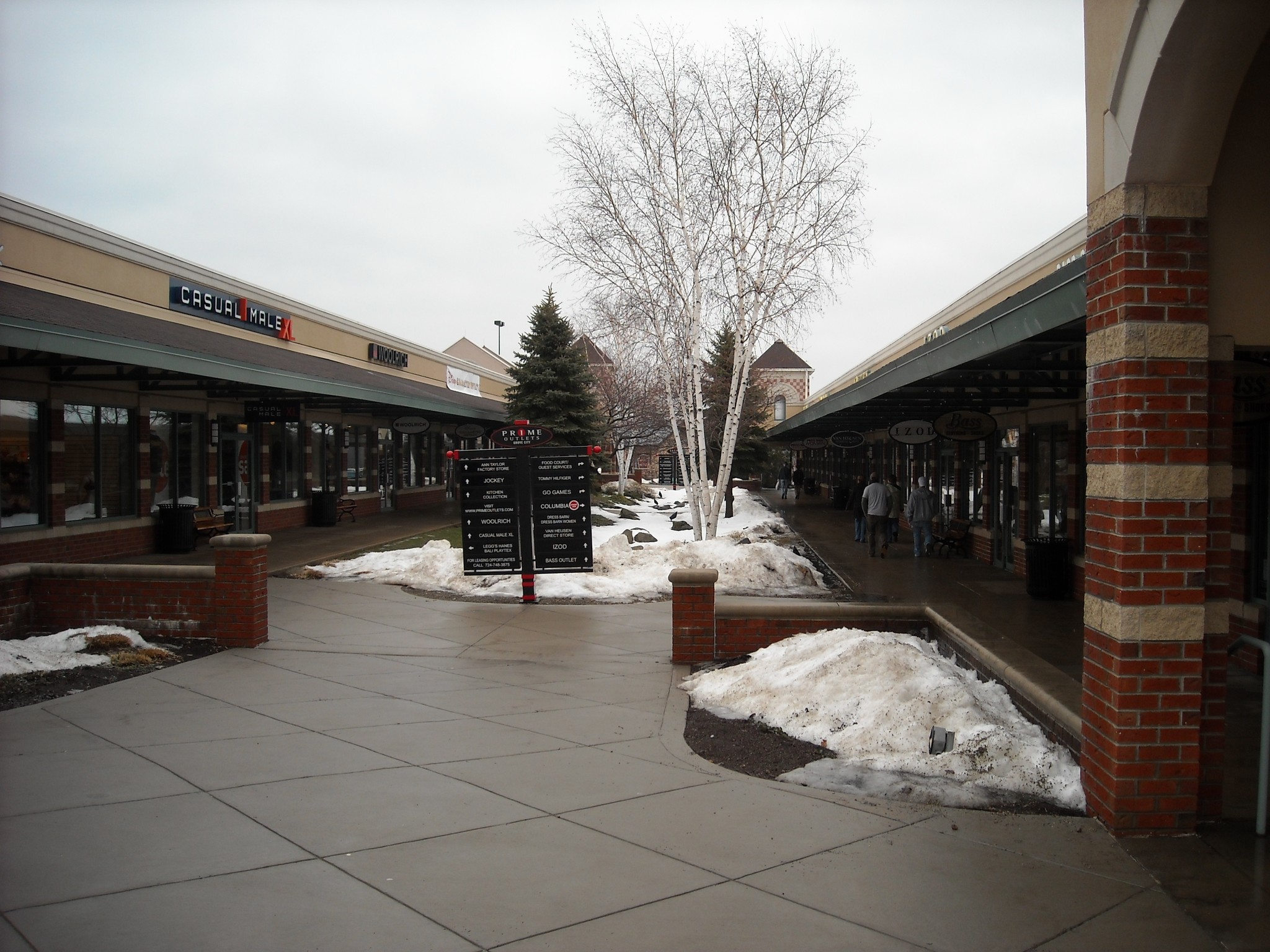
[193,507,233,540]
[929,518,970,558]
[335,497,358,523]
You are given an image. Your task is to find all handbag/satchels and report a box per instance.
[775,479,780,490]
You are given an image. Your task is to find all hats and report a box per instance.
[918,476,927,487]
[870,472,878,480]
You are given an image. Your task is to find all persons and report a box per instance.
[846,475,869,543]
[150,435,162,511]
[861,472,893,559]
[906,476,938,557]
[78,472,95,504]
[8,447,30,513]
[326,444,331,491]
[778,461,791,499]
[793,465,804,499]
[885,474,904,543]
[271,431,292,500]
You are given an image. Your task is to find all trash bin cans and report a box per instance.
[155,503,197,554]
[310,490,339,527]
[1018,536,1075,602]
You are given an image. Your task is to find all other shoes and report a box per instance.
[795,496,797,499]
[782,494,784,499]
[798,497,799,499]
[894,536,897,542]
[881,545,887,559]
[925,542,931,557]
[785,496,787,499]
[914,554,921,557]
